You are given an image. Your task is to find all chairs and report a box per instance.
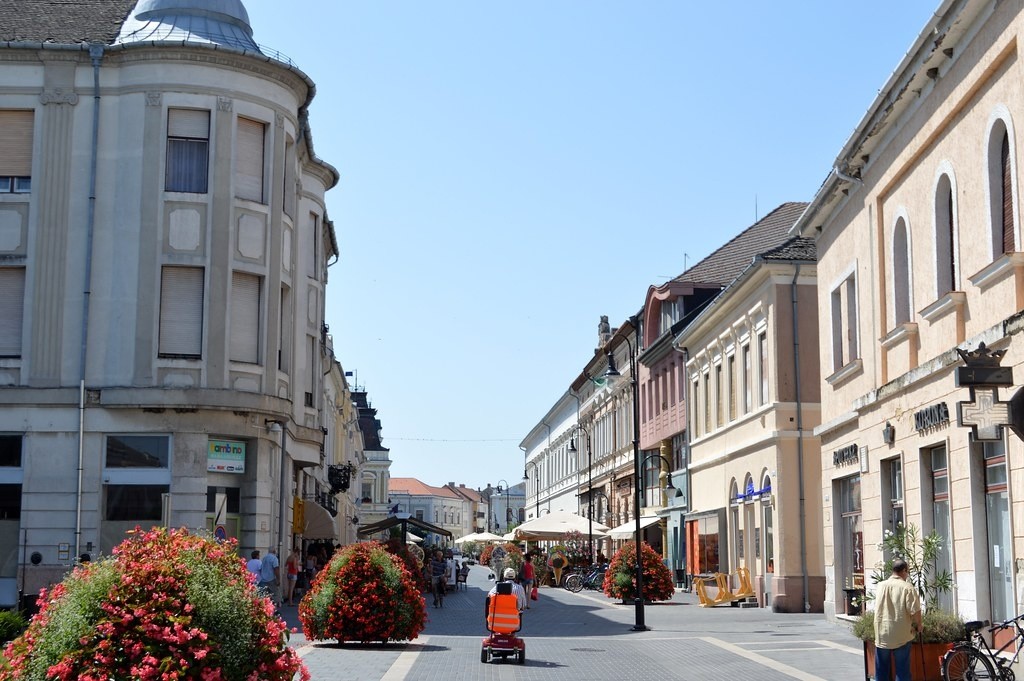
[485,582,523,634]
[456,568,470,591]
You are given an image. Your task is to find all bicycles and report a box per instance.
[561,562,609,593]
[428,574,444,609]
[938,613,1024,681]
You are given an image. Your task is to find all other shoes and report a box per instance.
[433,600,438,606]
[439,603,443,608]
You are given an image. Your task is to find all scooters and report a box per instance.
[481,581,526,665]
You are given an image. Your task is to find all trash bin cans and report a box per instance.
[843,588,864,615]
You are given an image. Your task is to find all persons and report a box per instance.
[874,560,923,681]
[284,546,302,607]
[518,554,537,609]
[597,549,609,572]
[260,546,280,603]
[430,550,448,607]
[488,567,527,611]
[304,542,342,589]
[455,559,468,575]
[247,550,262,585]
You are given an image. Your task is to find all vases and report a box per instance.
[864,638,972,681]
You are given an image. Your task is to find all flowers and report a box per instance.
[849,521,973,643]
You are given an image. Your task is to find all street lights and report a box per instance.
[522,461,550,563]
[601,331,677,631]
[567,427,613,570]
[496,479,509,544]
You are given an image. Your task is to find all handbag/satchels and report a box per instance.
[531,587,538,601]
[518,561,526,580]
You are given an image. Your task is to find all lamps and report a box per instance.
[265,418,283,433]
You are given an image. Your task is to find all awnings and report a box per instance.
[302,500,338,541]
[607,517,660,540]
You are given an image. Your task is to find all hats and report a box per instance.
[504,567,516,579]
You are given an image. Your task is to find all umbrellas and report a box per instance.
[455,531,504,543]
[503,511,611,541]
